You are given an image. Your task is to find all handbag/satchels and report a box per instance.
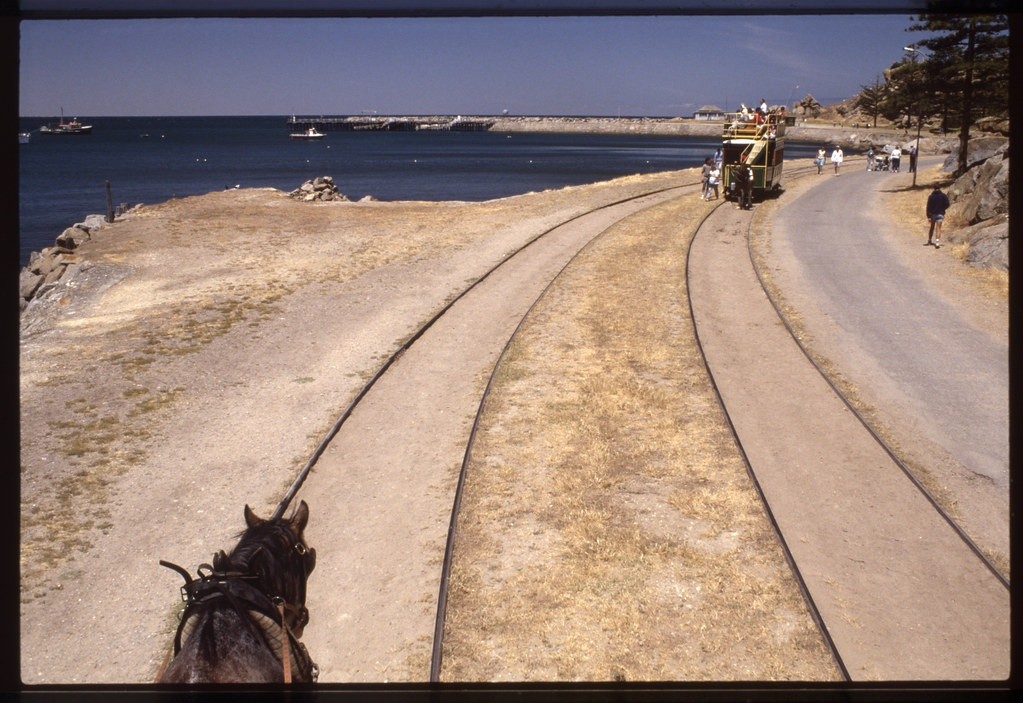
[814,159,817,164]
[700,175,704,183]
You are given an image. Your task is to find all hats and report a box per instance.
[836,145,840,148]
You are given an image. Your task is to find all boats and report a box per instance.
[40,107,93,133]
[19,131,31,144]
[289,127,329,138]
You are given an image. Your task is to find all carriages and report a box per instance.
[719,112,786,210]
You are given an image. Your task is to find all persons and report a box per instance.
[926,184,950,248]
[815,145,827,174]
[700,97,786,212]
[866,145,902,173]
[908,145,916,172]
[831,146,843,177]
[832,121,871,128]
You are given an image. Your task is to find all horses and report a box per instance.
[733,163,755,210]
[151,499,319,683]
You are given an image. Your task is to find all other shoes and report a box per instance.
[892,170,894,173]
[895,170,897,173]
[716,197,720,199]
[705,198,710,202]
[927,240,932,244]
[936,243,940,248]
[700,195,705,199]
